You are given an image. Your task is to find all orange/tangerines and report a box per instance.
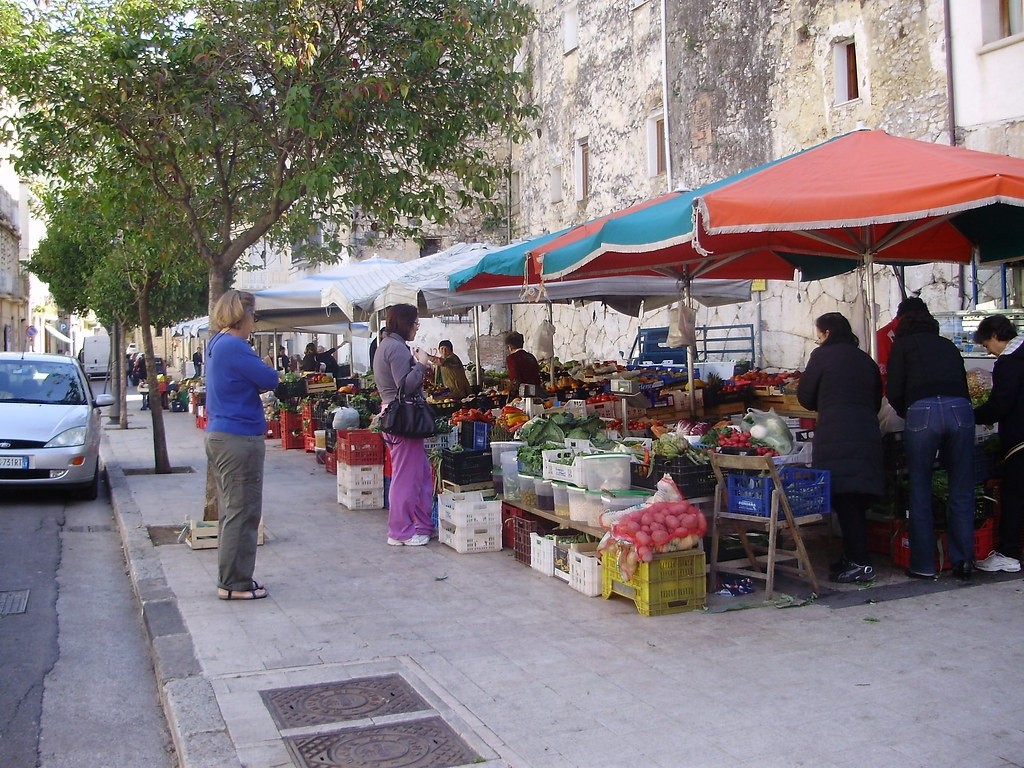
[735,371,782,387]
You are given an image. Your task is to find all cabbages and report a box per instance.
[524,412,607,444]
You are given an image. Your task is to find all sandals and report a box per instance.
[218,580,267,600]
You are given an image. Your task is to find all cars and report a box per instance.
[126,343,136,353]
[131,355,167,385]
[0,350,115,501]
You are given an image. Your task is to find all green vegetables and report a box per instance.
[276,370,381,432]
[882,467,1001,528]
[484,368,510,378]
[972,391,996,431]
[699,425,733,445]
[516,443,597,465]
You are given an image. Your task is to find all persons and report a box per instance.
[795,312,883,584]
[368,303,440,547]
[277,344,289,374]
[302,341,348,371]
[203,290,280,601]
[973,313,1023,573]
[878,295,978,581]
[505,331,541,403]
[317,345,338,378]
[263,349,274,368]
[426,340,472,397]
[193,347,203,378]
[125,353,147,387]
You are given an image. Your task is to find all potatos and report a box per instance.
[613,500,706,580]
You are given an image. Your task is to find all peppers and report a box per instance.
[494,406,530,432]
[451,408,496,425]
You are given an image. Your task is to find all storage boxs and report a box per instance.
[158,364,1024,615]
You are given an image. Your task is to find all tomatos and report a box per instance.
[605,418,653,430]
[586,392,619,406]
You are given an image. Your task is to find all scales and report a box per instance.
[279,358,289,376]
[610,379,651,437]
[518,383,539,420]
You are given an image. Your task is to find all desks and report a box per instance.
[684,494,804,579]
[496,494,605,541]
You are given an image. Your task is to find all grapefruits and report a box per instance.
[684,379,705,390]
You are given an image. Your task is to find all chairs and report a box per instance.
[0,372,9,391]
[707,449,821,601]
[40,372,81,402]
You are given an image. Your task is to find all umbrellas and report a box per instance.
[168,241,754,423]
[448,127,1023,424]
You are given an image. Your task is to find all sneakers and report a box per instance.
[428,531,438,538]
[973,550,1022,572]
[830,559,876,583]
[387,532,430,546]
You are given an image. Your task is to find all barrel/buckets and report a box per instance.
[315,446,326,464]
[314,430,326,448]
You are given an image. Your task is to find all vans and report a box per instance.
[81,336,110,377]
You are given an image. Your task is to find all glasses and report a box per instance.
[815,332,826,345]
[413,321,420,327]
[248,309,260,322]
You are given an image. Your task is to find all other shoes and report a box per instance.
[952,560,972,580]
[905,567,941,582]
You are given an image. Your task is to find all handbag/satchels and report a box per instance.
[740,407,793,455]
[331,406,360,430]
[377,376,438,444]
